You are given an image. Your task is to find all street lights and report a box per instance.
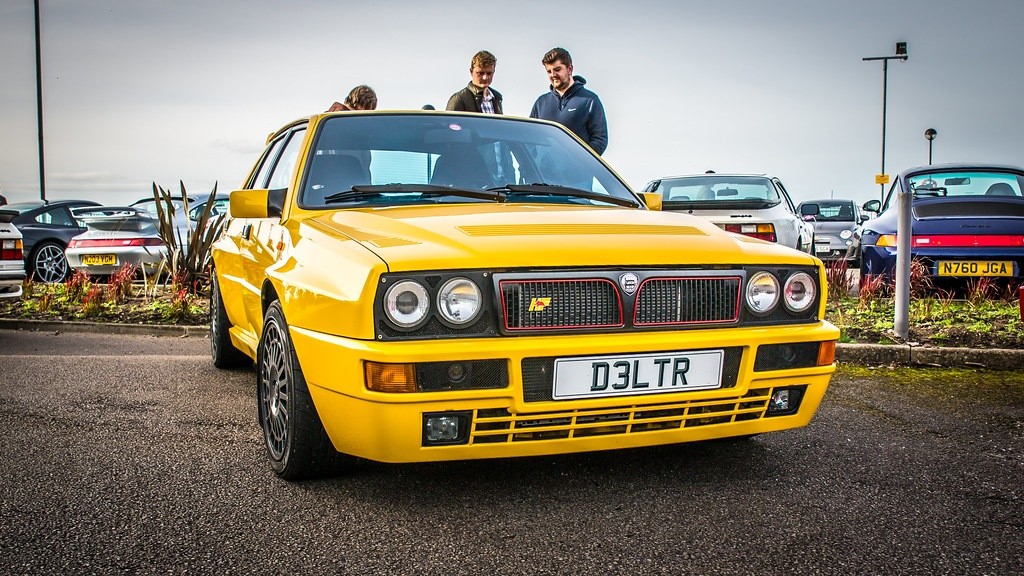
[863,42,908,213]
[923,128,937,186]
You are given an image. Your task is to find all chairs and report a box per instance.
[838,205,851,216]
[985,183,1017,196]
[668,196,690,201]
[421,149,496,197]
[913,185,938,198]
[301,154,367,208]
[194,206,213,221]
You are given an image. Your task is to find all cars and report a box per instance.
[860,163,1024,296]
[634,174,866,269]
[0,193,236,296]
[208,103,839,478]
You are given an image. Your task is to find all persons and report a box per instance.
[312,85,378,191]
[0,195,14,222]
[697,170,716,200]
[446,51,516,185]
[527,47,609,190]
